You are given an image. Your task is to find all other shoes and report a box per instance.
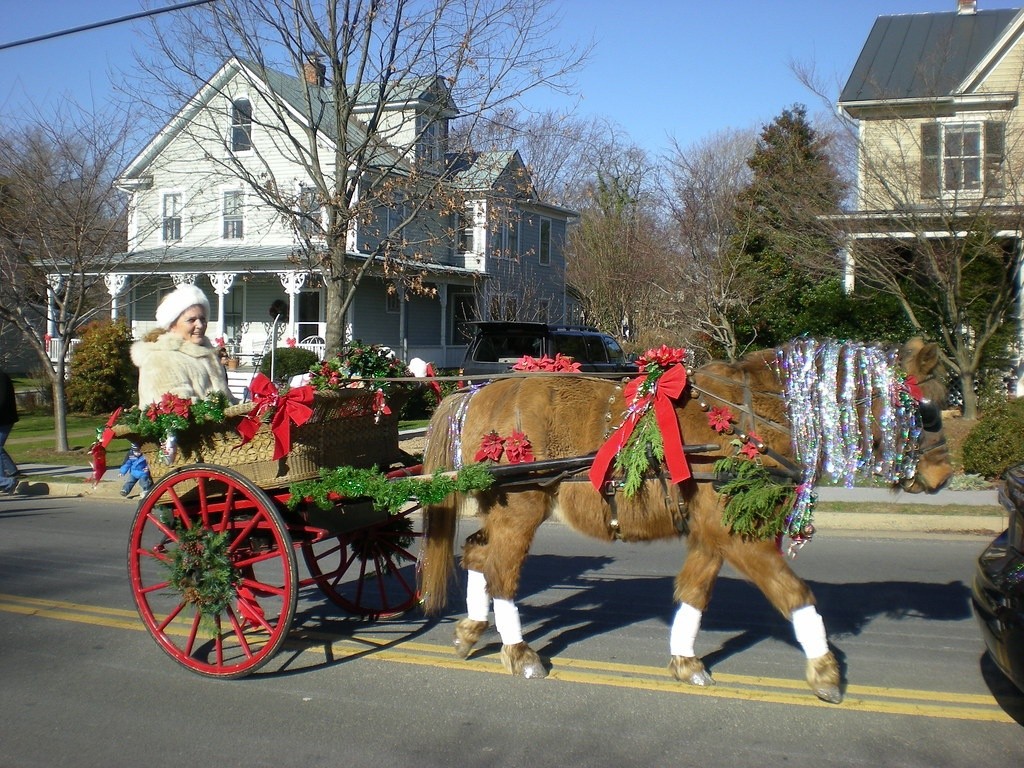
[120,489,127,497]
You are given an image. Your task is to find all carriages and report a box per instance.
[107,322,953,703]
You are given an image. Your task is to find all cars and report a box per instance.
[971,462,1024,696]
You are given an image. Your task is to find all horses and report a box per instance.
[416,337,952,705]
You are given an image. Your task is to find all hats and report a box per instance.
[131,442,139,451]
[156,283,210,327]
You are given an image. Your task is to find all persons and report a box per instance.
[0,365,20,495]
[130,284,237,412]
[217,351,228,385]
[118,443,153,498]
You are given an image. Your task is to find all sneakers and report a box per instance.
[0,474,29,498]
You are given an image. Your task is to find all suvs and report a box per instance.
[458,320,644,391]
